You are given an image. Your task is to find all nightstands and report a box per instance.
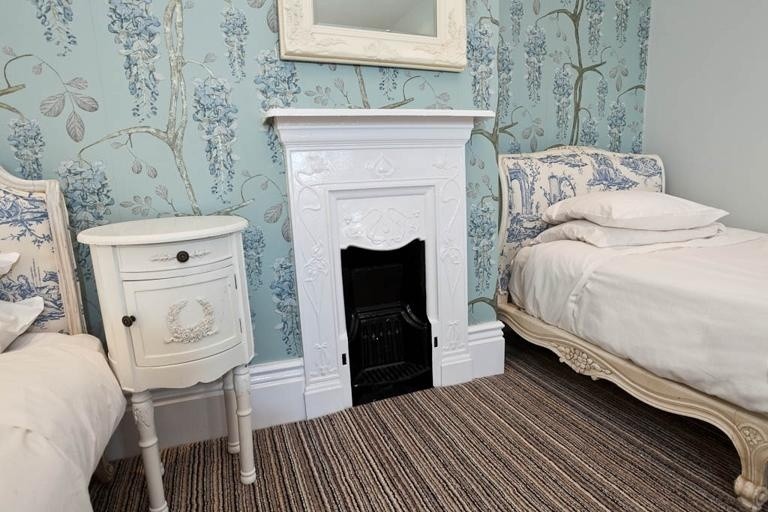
[77,215,257,511]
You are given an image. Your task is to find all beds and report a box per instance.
[494,144,764,510]
[0,165,128,512]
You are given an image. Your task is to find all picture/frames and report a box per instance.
[277,0,471,75]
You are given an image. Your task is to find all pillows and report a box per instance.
[525,222,729,250]
[0,298,45,355]
[538,188,731,228]
[0,253,23,280]
